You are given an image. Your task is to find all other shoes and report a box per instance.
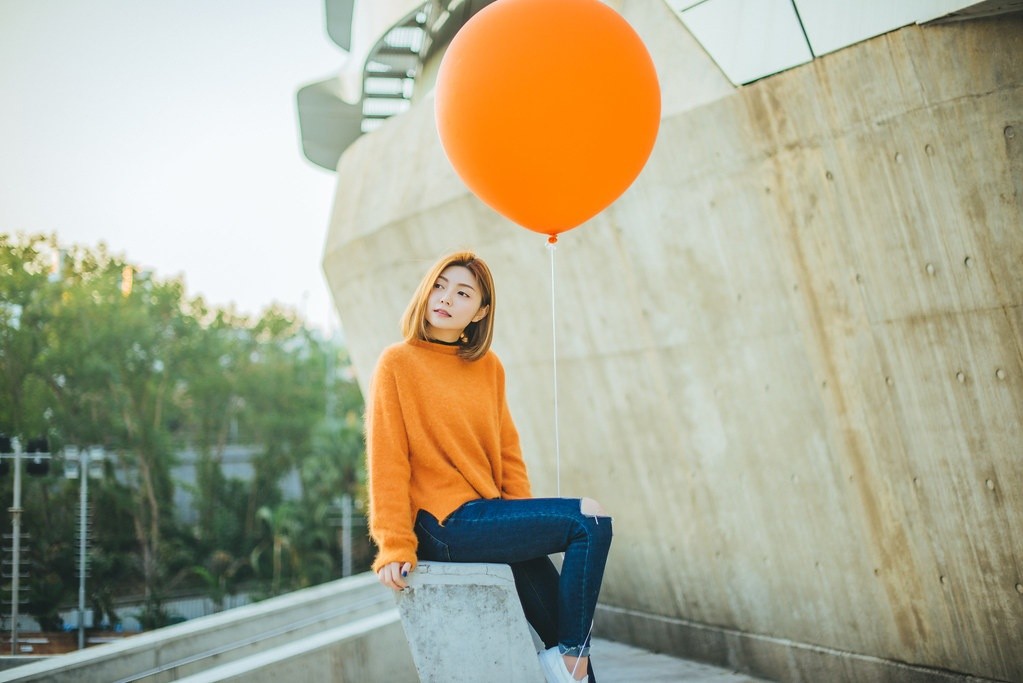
[539,645,589,683]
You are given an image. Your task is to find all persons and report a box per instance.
[364,251,613,683]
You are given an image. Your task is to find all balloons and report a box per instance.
[436,0,661,241]
